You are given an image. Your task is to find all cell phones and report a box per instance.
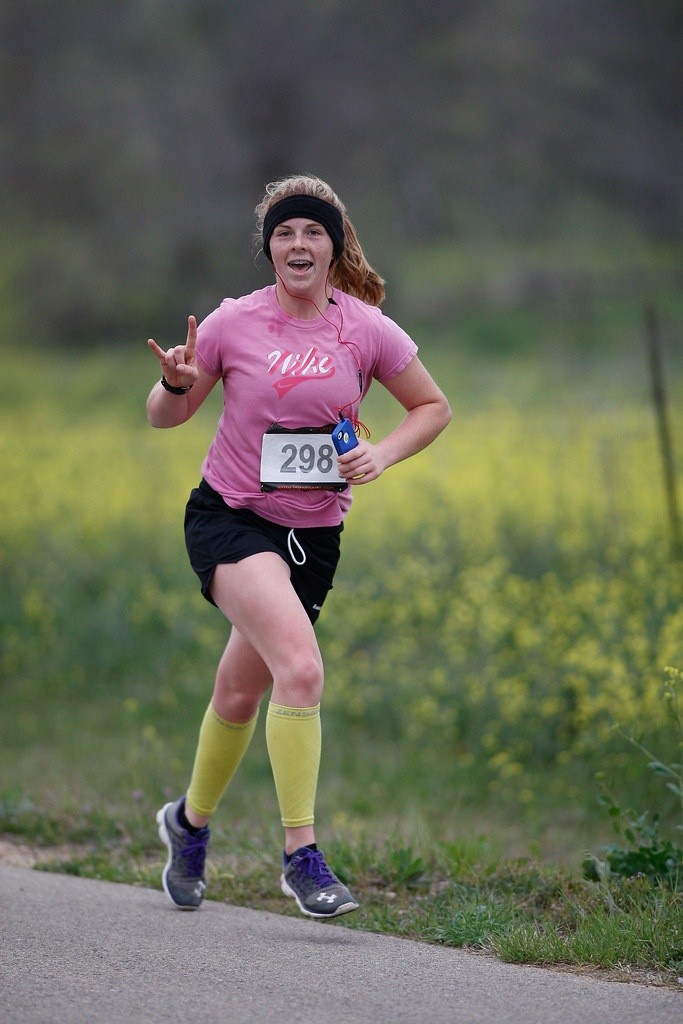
[331,417,360,456]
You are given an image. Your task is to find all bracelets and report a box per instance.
[161,375,193,395]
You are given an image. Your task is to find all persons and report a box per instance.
[145,178,453,916]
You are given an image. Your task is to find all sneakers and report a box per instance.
[280,848,359,918]
[156,796,209,911]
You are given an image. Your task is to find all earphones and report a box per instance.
[328,258,336,269]
